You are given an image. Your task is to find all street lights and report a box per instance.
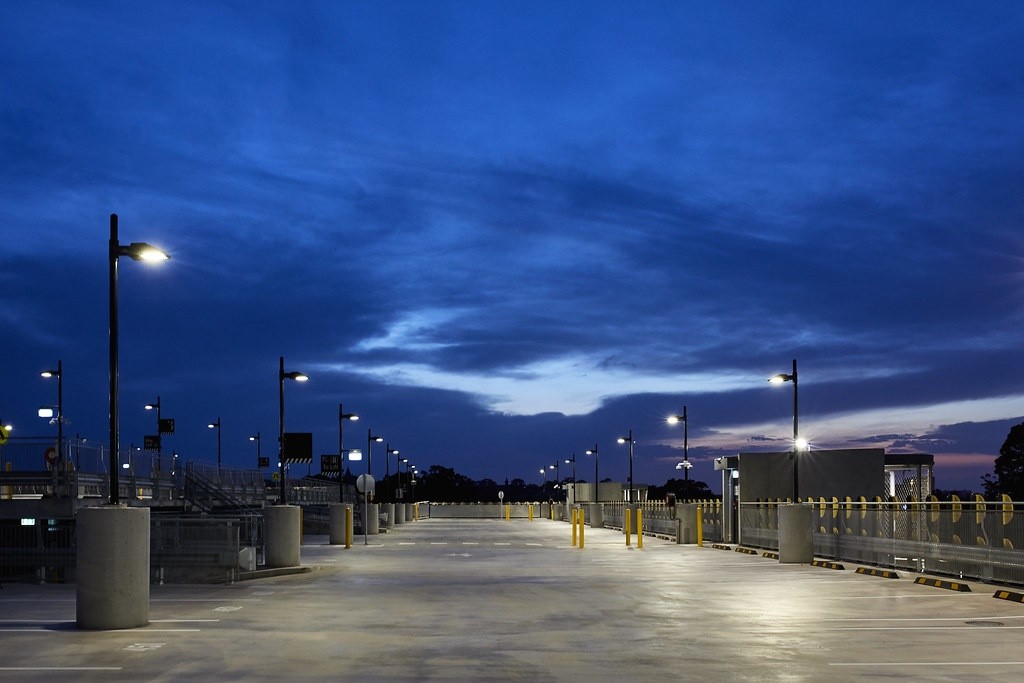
[540,469,546,485]
[109,240,170,502]
[549,465,560,502]
[279,370,310,504]
[144,403,161,473]
[767,373,807,502]
[618,438,633,504]
[586,450,598,503]
[386,449,399,503]
[397,458,419,503]
[250,436,261,469]
[338,414,361,503]
[208,423,220,475]
[565,459,576,504]
[38,370,63,456]
[367,436,384,475]
[668,415,692,503]
